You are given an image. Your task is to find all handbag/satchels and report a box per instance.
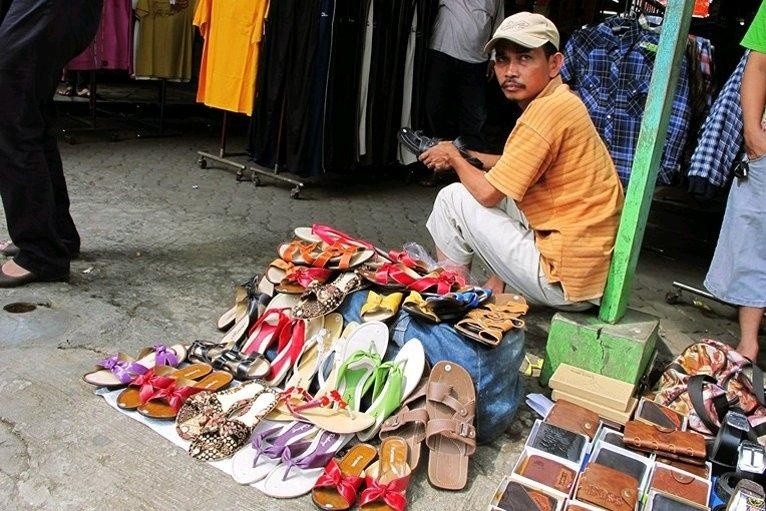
[652,338,766,435]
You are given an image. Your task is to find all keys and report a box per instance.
[734,161,749,179]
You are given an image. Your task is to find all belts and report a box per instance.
[710,411,766,511]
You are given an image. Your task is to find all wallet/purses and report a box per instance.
[498,400,709,511]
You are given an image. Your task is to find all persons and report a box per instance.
[703,0,766,365]
[0,0,103,288]
[417,0,505,188]
[417,12,625,311]
[56,68,91,97]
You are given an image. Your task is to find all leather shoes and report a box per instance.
[0,241,80,287]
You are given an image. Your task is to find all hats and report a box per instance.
[484,11,560,53]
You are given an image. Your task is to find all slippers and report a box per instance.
[81,338,217,461]
[397,126,483,173]
[266,224,529,349]
[218,275,427,511]
[426,360,476,490]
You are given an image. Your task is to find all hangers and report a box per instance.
[589,0,671,25]
